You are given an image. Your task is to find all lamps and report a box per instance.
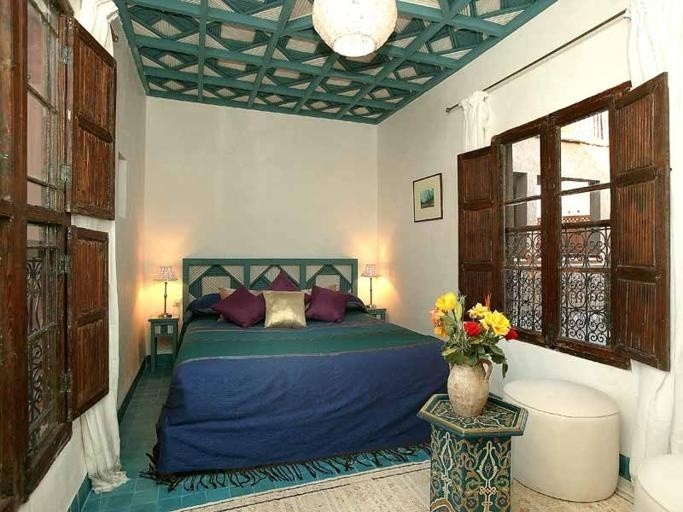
[151,265,179,316]
[362,264,379,306]
[310,0,397,59]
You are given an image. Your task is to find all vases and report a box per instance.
[449,359,492,417]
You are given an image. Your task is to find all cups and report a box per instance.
[172,305,179,319]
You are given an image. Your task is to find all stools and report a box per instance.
[636,457,683,510]
[503,379,621,501]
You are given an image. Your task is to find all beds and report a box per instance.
[138,259,448,491]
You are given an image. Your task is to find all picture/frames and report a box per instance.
[412,172,442,223]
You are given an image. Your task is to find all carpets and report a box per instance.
[76,355,438,512]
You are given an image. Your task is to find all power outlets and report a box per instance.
[172,299,181,308]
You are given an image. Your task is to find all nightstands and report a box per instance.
[149,316,179,372]
[366,304,386,321]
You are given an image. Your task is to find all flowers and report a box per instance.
[431,289,518,378]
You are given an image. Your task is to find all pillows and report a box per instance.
[306,284,350,323]
[213,286,263,327]
[218,286,260,324]
[303,284,337,313]
[264,290,307,329]
[270,270,300,290]
[346,294,365,309]
[185,293,221,312]
